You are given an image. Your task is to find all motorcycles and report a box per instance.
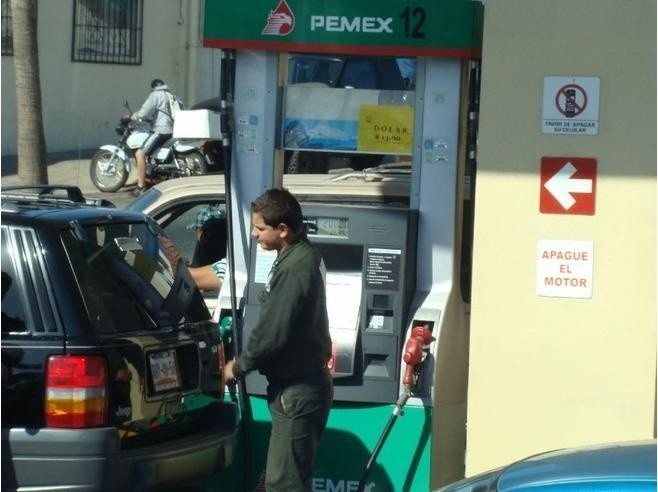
[88,99,206,191]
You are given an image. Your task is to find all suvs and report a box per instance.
[1,184,239,491]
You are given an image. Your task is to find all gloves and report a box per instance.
[122,112,131,125]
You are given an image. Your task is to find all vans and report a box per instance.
[122,166,471,323]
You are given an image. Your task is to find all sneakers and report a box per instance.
[128,185,148,197]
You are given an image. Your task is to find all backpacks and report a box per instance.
[155,90,185,121]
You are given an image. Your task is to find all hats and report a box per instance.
[184,207,227,231]
[150,78,163,89]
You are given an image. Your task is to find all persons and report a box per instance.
[120,78,185,197]
[221,189,337,491]
[179,206,229,292]
